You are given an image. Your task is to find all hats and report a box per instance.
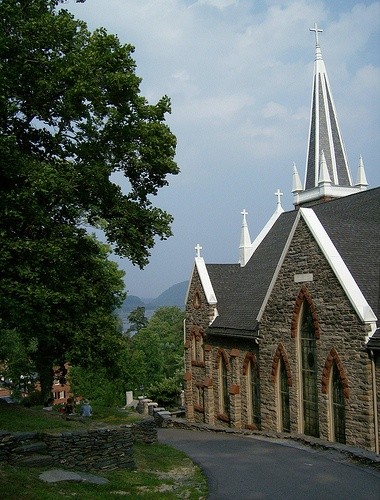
[83,399,89,404]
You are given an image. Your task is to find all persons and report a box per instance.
[81,399,93,417]
[64,397,77,414]
[44,398,54,409]
